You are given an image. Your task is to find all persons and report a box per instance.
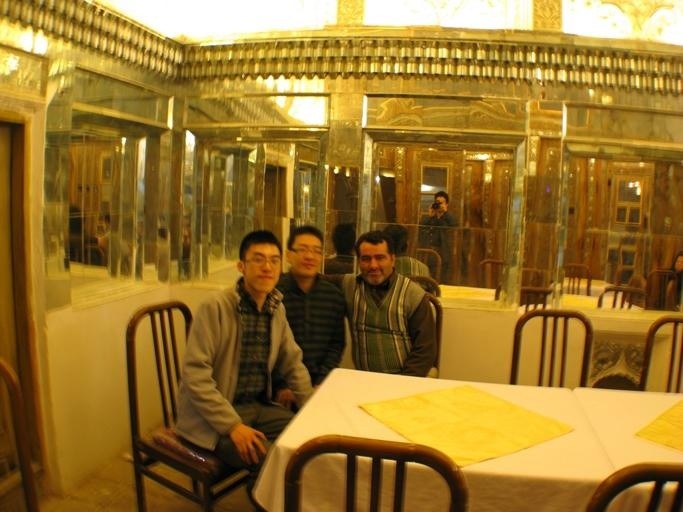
[418,190,456,284]
[323,224,356,274]
[381,225,430,277]
[175,228,314,512]
[320,229,436,376]
[275,228,344,388]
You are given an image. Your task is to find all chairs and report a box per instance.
[123,300,259,512]
[636,315,683,393]
[70,214,190,272]
[509,309,593,394]
[582,460,683,512]
[281,433,470,511]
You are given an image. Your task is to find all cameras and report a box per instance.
[433,200,440,209]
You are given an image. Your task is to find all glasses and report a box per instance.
[290,247,323,258]
[242,256,281,267]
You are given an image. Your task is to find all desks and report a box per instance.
[270,364,683,511]
